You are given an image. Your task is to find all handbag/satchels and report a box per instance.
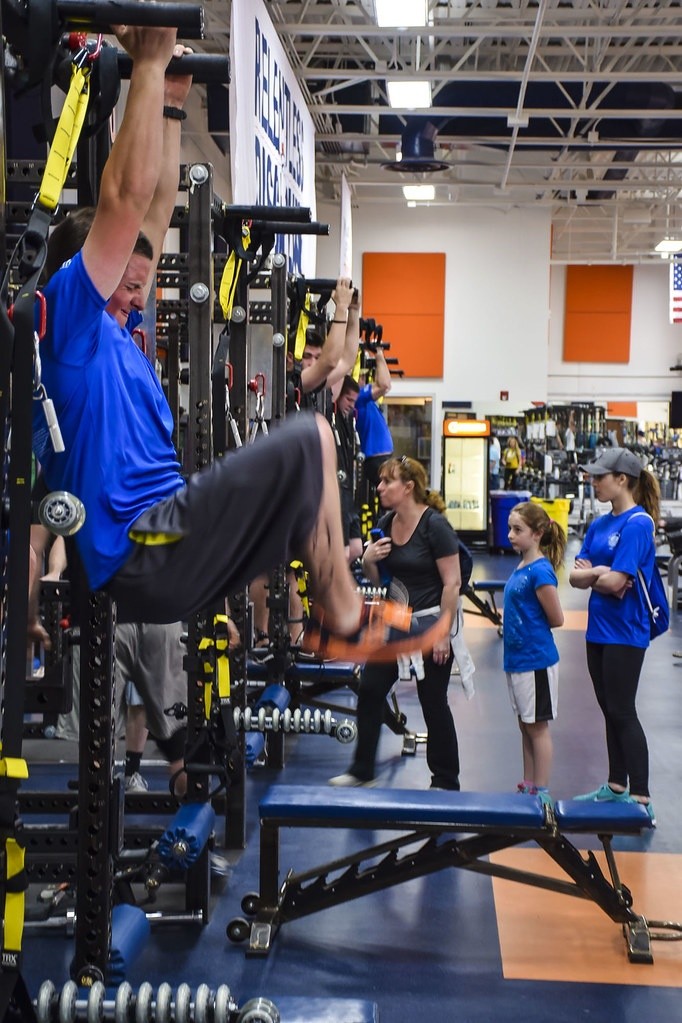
[515,473,523,485]
[628,512,670,642]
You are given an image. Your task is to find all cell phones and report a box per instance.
[371,529,385,543]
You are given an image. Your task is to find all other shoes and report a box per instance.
[532,791,553,808]
[515,782,534,794]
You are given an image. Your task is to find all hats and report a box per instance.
[578,448,642,477]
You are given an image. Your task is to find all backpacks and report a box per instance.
[386,508,473,596]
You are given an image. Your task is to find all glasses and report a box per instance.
[397,455,407,470]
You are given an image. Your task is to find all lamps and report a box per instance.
[386,79,433,110]
[655,237,682,253]
[404,186,435,202]
[375,1,427,27]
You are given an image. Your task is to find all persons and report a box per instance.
[249,278,394,650]
[503,436,523,490]
[553,418,575,450]
[502,502,564,797]
[569,446,662,823]
[54,617,240,793]
[489,432,501,489]
[6,27,451,659]
[329,457,462,790]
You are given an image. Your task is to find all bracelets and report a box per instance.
[163,105,187,121]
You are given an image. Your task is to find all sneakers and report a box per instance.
[328,773,378,788]
[124,772,148,792]
[299,597,454,665]
[573,784,629,803]
[622,797,656,825]
[430,787,447,790]
